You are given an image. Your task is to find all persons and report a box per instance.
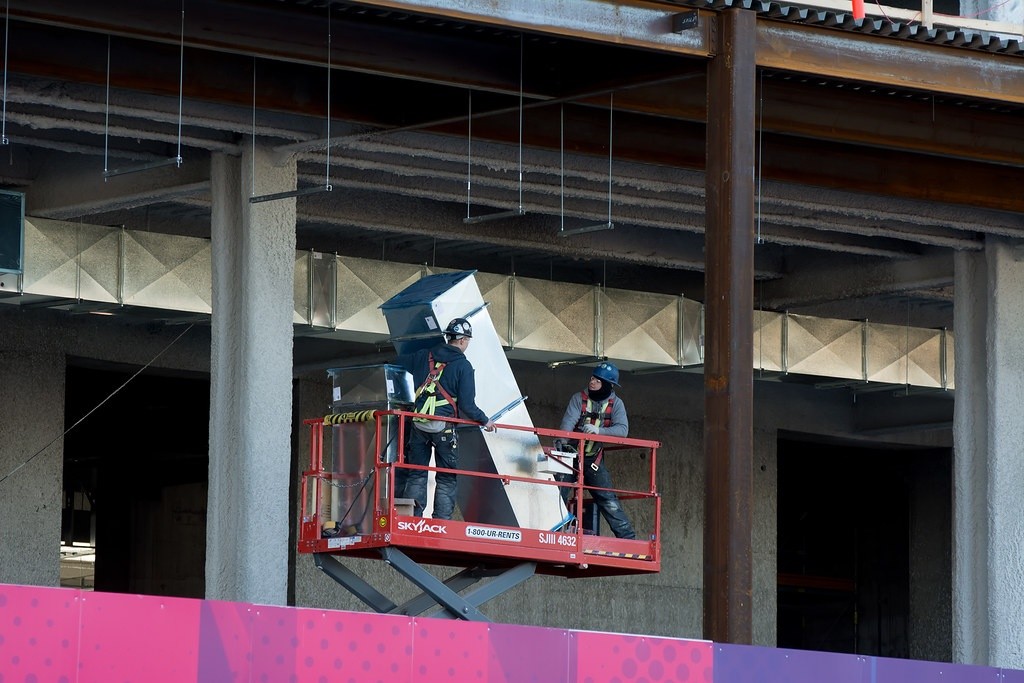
[553,364,640,542]
[383,318,497,522]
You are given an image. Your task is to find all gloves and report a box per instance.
[583,424,598,434]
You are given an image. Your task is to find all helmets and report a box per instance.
[443,319,473,337]
[593,362,621,387]
